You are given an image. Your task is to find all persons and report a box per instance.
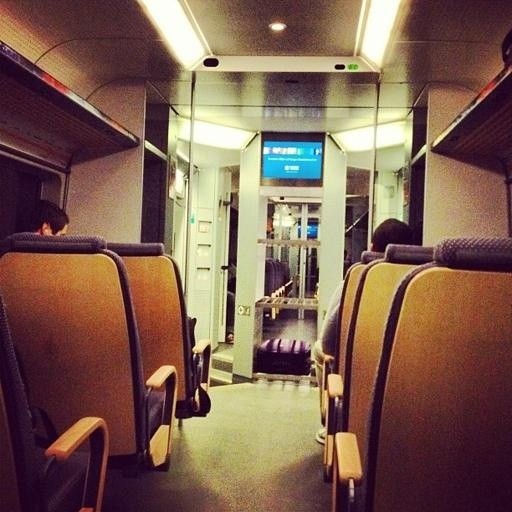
[16,199,71,237]
[322,218,418,355]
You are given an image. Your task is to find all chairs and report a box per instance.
[1,230,183,478]
[320,241,438,498]
[1,400,114,512]
[328,239,511,510]
[313,241,389,461]
[97,239,212,430]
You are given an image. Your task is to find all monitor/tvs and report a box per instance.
[298,221,318,238]
[261,132,325,187]
